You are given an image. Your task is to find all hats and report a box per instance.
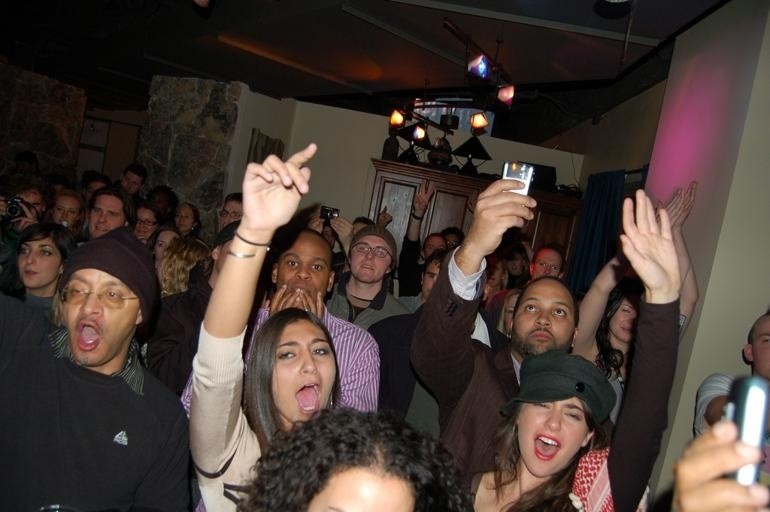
[353,226,397,267]
[499,350,615,418]
[59,226,160,327]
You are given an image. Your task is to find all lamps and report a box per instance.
[391,19,515,152]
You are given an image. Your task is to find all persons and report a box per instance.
[0,141,769,510]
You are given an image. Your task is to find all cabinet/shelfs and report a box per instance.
[368,157,584,277]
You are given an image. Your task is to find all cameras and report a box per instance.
[320,206,339,220]
[2,197,31,219]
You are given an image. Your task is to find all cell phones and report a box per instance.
[733,376,770,486]
[501,161,534,196]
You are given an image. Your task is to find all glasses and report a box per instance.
[350,241,391,259]
[60,282,142,308]
[534,260,561,272]
[219,209,242,218]
